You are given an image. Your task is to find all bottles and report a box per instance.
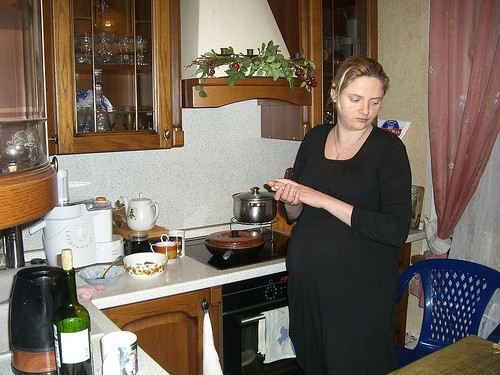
[88,69,111,132]
[52,249,94,375]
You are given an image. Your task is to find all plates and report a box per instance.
[76,89,114,132]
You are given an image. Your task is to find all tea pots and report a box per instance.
[121,194,160,233]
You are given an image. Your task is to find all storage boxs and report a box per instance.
[113,223,168,255]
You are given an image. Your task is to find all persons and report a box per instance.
[267,55,412,374]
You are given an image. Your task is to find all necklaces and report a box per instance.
[334,122,370,160]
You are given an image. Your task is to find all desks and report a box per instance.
[389,334,500,375]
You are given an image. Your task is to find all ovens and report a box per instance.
[222,271,307,375]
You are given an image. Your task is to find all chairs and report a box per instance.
[393,258,500,369]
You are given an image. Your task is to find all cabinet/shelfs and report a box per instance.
[393,242,412,347]
[182,48,314,142]
[42,0,184,156]
[265,0,378,127]
[100,286,220,375]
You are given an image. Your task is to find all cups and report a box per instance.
[74,33,149,66]
[101,331,138,375]
[151,241,178,264]
[161,230,185,257]
[128,232,150,256]
[138,105,153,131]
[121,106,135,130]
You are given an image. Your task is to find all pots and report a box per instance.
[205,229,267,260]
[232,187,277,223]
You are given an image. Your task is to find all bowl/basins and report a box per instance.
[77,107,119,134]
[78,265,125,286]
[123,253,170,277]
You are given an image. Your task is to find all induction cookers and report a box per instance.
[181,228,291,271]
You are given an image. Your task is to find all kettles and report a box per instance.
[8,266,72,374]
